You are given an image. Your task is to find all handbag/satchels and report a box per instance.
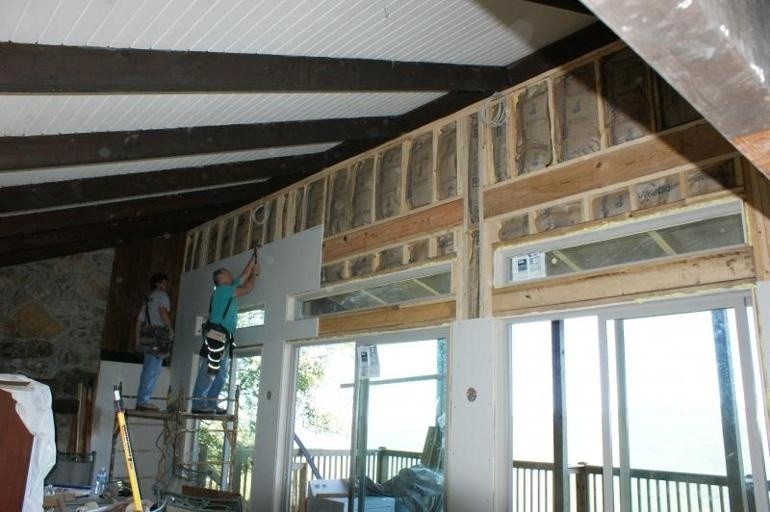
[140,326,171,345]
[201,322,228,359]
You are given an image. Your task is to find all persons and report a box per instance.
[134,271,174,410]
[192,254,259,414]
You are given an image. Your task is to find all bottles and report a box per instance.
[45,485,56,497]
[94,468,107,497]
